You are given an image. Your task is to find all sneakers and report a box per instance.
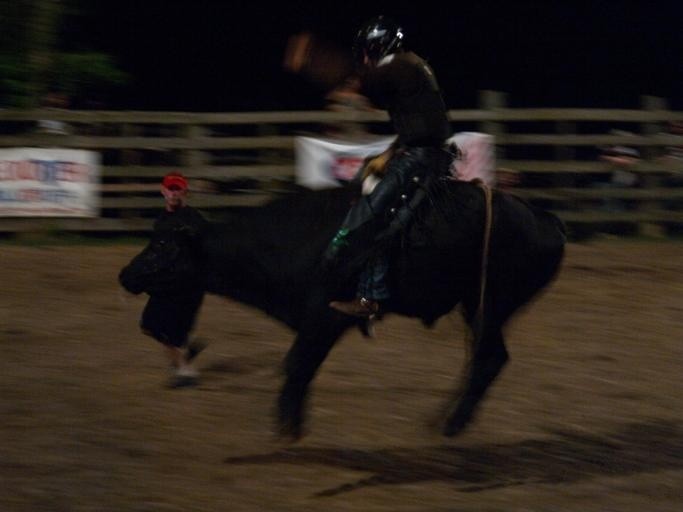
[325,294,378,324]
[156,338,205,388]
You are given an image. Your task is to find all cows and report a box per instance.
[118,177,567,440]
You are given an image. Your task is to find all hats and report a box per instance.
[161,172,190,192]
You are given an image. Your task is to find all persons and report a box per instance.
[136,172,213,390]
[288,14,450,326]
[652,117,683,234]
[36,82,81,147]
[322,79,389,140]
[164,122,225,194]
[597,126,644,231]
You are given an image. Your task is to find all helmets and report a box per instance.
[351,16,409,61]
